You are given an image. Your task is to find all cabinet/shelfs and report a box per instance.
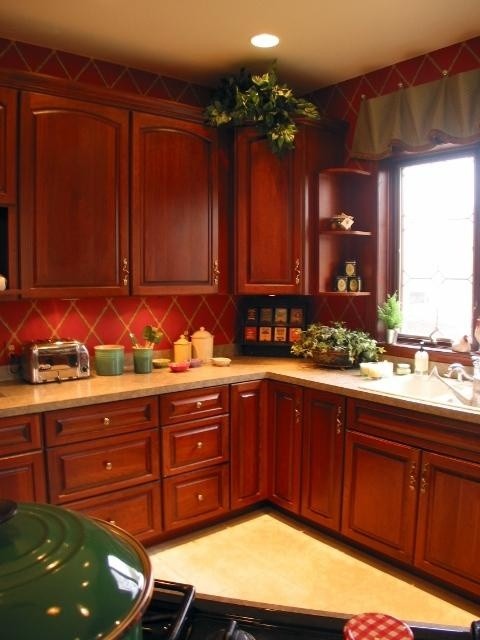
[43,396,164,544]
[0,64,21,302]
[341,394,480,602]
[234,118,344,296]
[315,166,375,297]
[265,374,346,533]
[0,415,47,505]
[20,67,218,297]
[162,385,232,539]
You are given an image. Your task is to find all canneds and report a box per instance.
[333,275,348,291]
[348,276,361,292]
[345,260,357,278]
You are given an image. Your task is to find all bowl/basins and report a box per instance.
[152,359,171,367]
[210,357,231,366]
[190,359,202,368]
[170,363,190,372]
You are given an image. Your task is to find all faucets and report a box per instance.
[443,356,480,409]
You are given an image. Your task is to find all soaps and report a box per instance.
[397,362,410,370]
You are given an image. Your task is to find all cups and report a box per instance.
[133,348,152,374]
[173,334,191,362]
[95,345,123,375]
[190,326,213,363]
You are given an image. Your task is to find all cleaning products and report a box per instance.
[412,341,430,373]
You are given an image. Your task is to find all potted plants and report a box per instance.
[377,289,402,345]
[290,319,385,370]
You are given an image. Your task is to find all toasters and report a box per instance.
[21,339,90,384]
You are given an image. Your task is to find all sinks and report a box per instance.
[357,373,480,415]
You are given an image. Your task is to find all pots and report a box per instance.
[0,499,194,640]
